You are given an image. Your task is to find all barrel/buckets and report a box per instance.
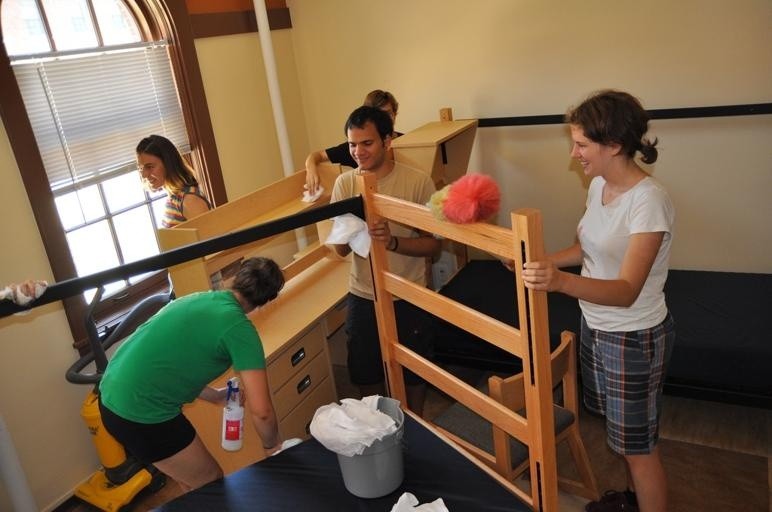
[336,395,406,499]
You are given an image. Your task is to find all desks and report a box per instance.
[420,329,601,503]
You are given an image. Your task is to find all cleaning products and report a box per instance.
[220,376,247,454]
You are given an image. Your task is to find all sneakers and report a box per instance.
[584,488,638,512]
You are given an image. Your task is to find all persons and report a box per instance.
[499,89,676,512]
[304,89,407,196]
[134,133,212,230]
[327,108,441,422]
[97,258,285,494]
